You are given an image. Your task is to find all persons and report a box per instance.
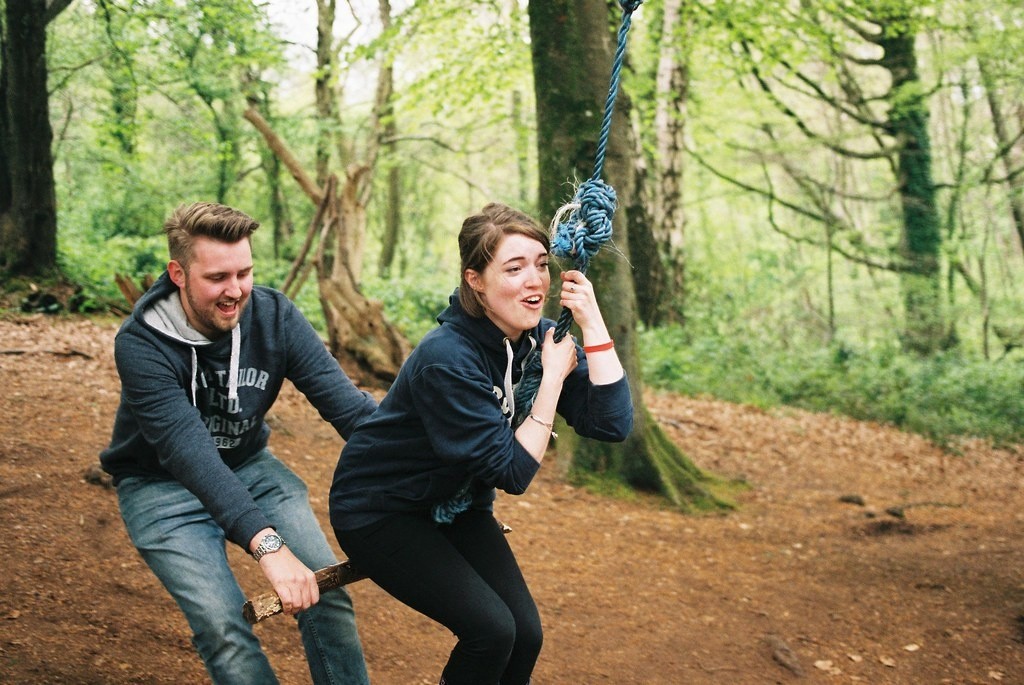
[99,204,381,685]
[330,203,633,685]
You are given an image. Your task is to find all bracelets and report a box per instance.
[530,412,558,440]
[584,339,615,353]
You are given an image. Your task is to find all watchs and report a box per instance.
[254,534,286,562]
